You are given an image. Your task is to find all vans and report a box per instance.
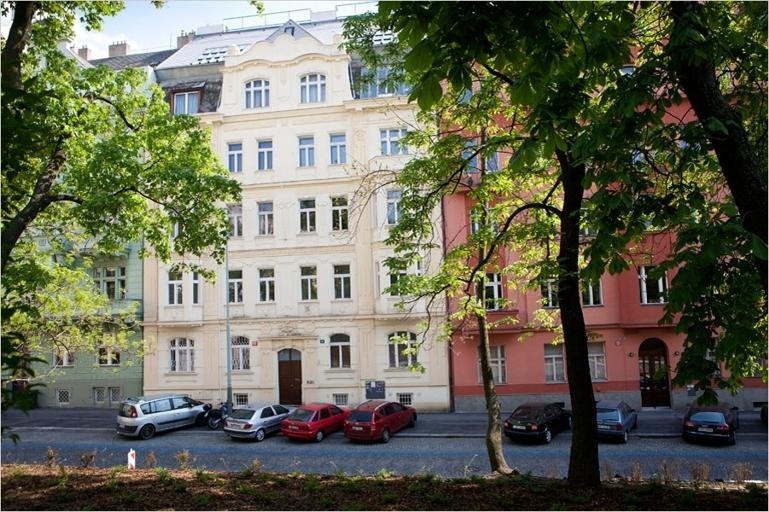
[116,392,209,439]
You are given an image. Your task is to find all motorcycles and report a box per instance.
[203,400,237,429]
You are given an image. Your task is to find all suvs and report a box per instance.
[344,401,416,445]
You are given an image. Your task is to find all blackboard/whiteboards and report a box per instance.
[365,380,385,399]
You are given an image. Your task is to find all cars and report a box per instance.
[224,404,299,441]
[682,400,739,446]
[597,400,637,443]
[281,402,351,443]
[503,401,573,444]
